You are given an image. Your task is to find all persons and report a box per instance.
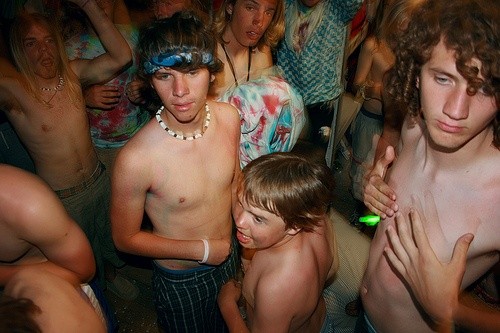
[0,1,140,302]
[111,18,242,333]
[0,162,113,333]
[345,0,500,333]
[153,0,191,20]
[217,152,338,333]
[208,0,273,104]
[276,0,364,149]
[0,268,108,333]
[347,1,428,229]
[64,0,153,175]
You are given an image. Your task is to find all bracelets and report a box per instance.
[197,239,209,264]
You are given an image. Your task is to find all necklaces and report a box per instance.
[219,37,252,87]
[39,73,63,91]
[156,103,211,140]
